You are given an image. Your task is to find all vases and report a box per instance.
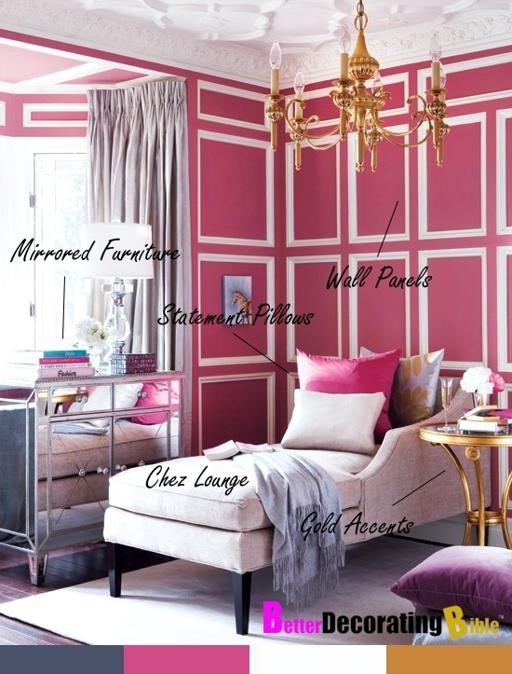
[461,367,506,396]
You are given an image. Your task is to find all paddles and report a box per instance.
[0,535,445,645]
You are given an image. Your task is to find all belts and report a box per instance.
[438,377,456,431]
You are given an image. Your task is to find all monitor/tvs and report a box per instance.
[10,348,93,387]
[202,440,274,461]
[459,404,500,433]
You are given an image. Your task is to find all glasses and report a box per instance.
[263,1,451,173]
[79,221,155,373]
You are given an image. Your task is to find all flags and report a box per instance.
[470,392,491,407]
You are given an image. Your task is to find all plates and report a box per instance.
[0,372,193,585]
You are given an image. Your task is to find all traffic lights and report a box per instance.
[131,381,180,425]
[280,345,446,456]
[389,542,512,623]
[81,383,144,429]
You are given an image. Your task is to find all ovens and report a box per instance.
[102,376,493,635]
